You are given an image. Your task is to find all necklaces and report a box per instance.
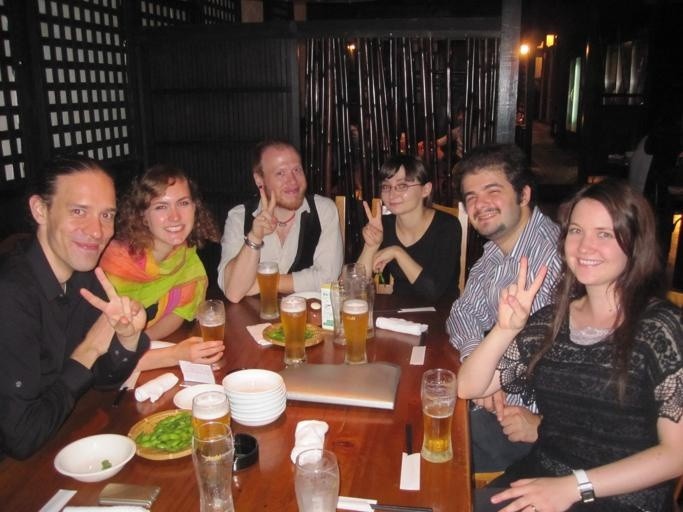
[273,212,296,228]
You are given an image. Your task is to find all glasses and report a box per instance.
[381,183,423,192]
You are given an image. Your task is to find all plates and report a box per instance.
[126,408,197,462]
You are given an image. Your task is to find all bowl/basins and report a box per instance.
[54,433,138,484]
[166,369,289,427]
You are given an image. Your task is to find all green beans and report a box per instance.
[135,412,195,453]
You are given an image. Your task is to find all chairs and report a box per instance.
[371,198,469,297]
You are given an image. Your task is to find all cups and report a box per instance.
[293,448,341,512]
[194,261,375,366]
[418,368,457,463]
[188,390,236,512]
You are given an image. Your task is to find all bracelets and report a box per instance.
[242,234,266,249]
[572,467,597,505]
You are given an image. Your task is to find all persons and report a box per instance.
[455,177,683,512]
[355,153,463,302]
[1,146,152,464]
[347,103,467,207]
[96,160,227,372]
[444,142,564,474]
[219,136,347,304]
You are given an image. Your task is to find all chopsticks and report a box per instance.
[404,422,414,454]
[419,331,426,347]
[112,386,127,408]
[369,504,434,512]
